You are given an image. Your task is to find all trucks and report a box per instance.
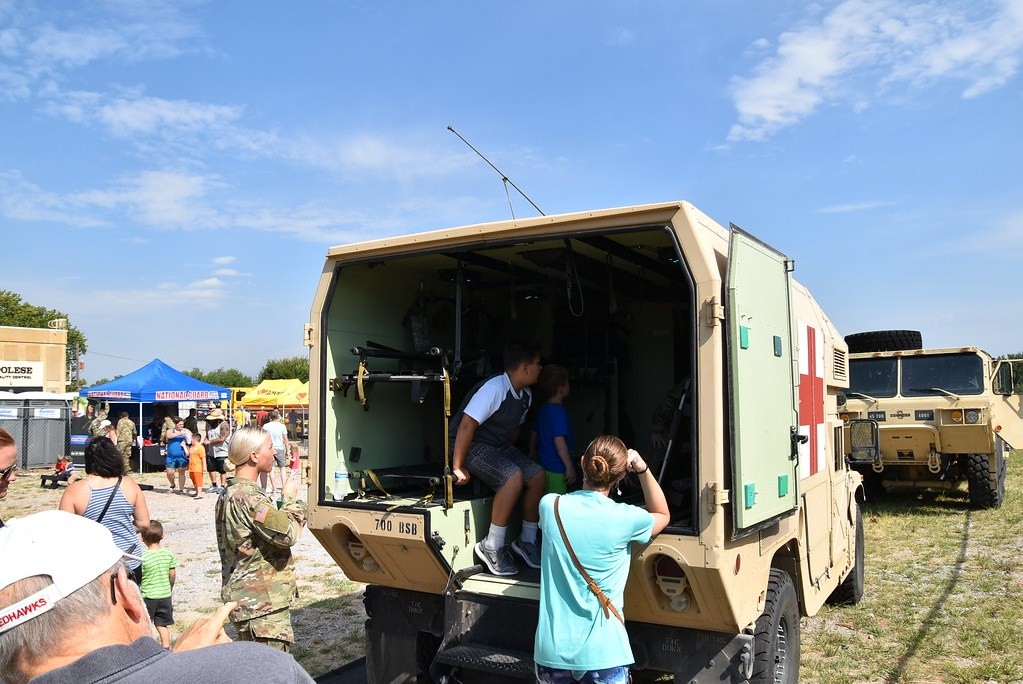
[838,345,1023,511]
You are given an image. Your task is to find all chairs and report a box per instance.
[849,364,879,392]
[930,359,969,389]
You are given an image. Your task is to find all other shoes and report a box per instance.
[194,495,203,499]
[206,486,218,493]
[216,488,224,494]
[272,491,277,495]
[191,493,197,497]
[179,490,184,493]
[167,484,176,493]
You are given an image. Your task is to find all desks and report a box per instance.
[131,445,166,472]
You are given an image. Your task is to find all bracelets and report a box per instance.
[637,463,648,474]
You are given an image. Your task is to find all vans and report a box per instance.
[248,409,309,438]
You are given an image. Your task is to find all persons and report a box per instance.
[0,425,317,684]
[533,433,671,684]
[48,399,303,500]
[447,334,577,576]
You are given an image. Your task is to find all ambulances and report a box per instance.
[307,192,870,682]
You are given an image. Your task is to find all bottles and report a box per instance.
[160,441,166,455]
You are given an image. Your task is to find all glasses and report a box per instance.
[0,464,18,481]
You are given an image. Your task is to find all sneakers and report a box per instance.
[475,536,518,576]
[511,538,541,568]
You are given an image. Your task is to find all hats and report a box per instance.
[0,510,125,636]
[206,409,226,421]
[99,420,111,429]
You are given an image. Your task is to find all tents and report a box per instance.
[78,358,309,475]
